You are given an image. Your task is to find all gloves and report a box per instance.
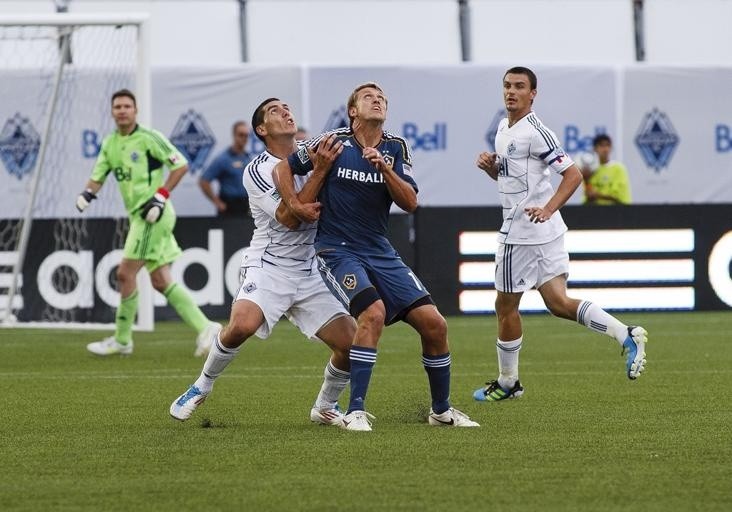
[139,188,169,224]
[75,188,97,212]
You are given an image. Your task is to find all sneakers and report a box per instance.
[169,385,212,421]
[86,336,133,356]
[193,322,223,357]
[429,407,480,427]
[620,326,648,379]
[310,400,373,432]
[473,379,524,401]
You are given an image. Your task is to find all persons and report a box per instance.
[577,133,633,206]
[199,121,253,217]
[472,66,649,402]
[292,127,308,141]
[271,81,482,433]
[76,87,224,358]
[168,97,359,431]
